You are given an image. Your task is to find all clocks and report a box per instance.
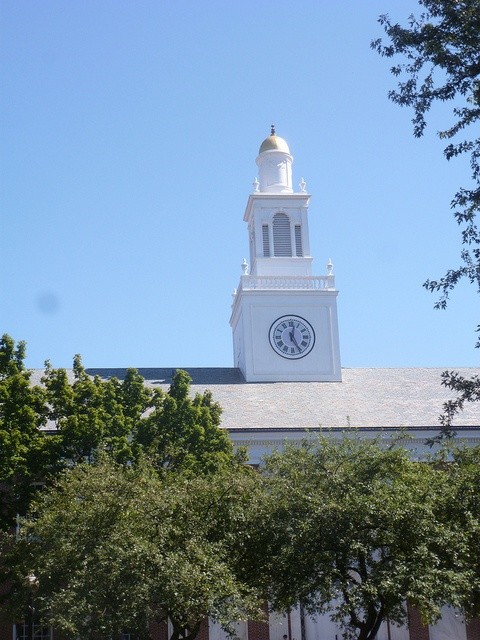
[268,315,317,359]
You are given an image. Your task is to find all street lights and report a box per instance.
[24,569,40,640]
